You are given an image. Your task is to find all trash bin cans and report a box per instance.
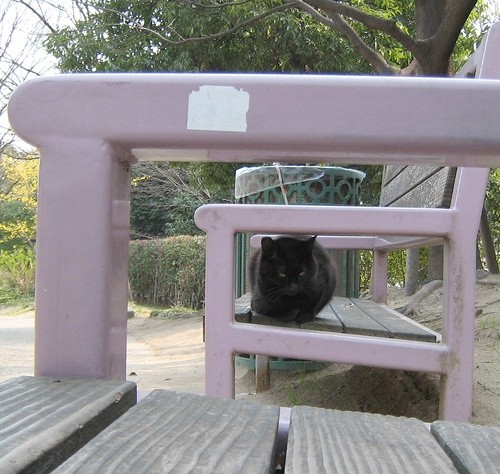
[234,165,367,373]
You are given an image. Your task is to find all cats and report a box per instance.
[247,233,337,323]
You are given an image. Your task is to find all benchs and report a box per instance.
[1,69,500,474]
[192,19,500,423]
